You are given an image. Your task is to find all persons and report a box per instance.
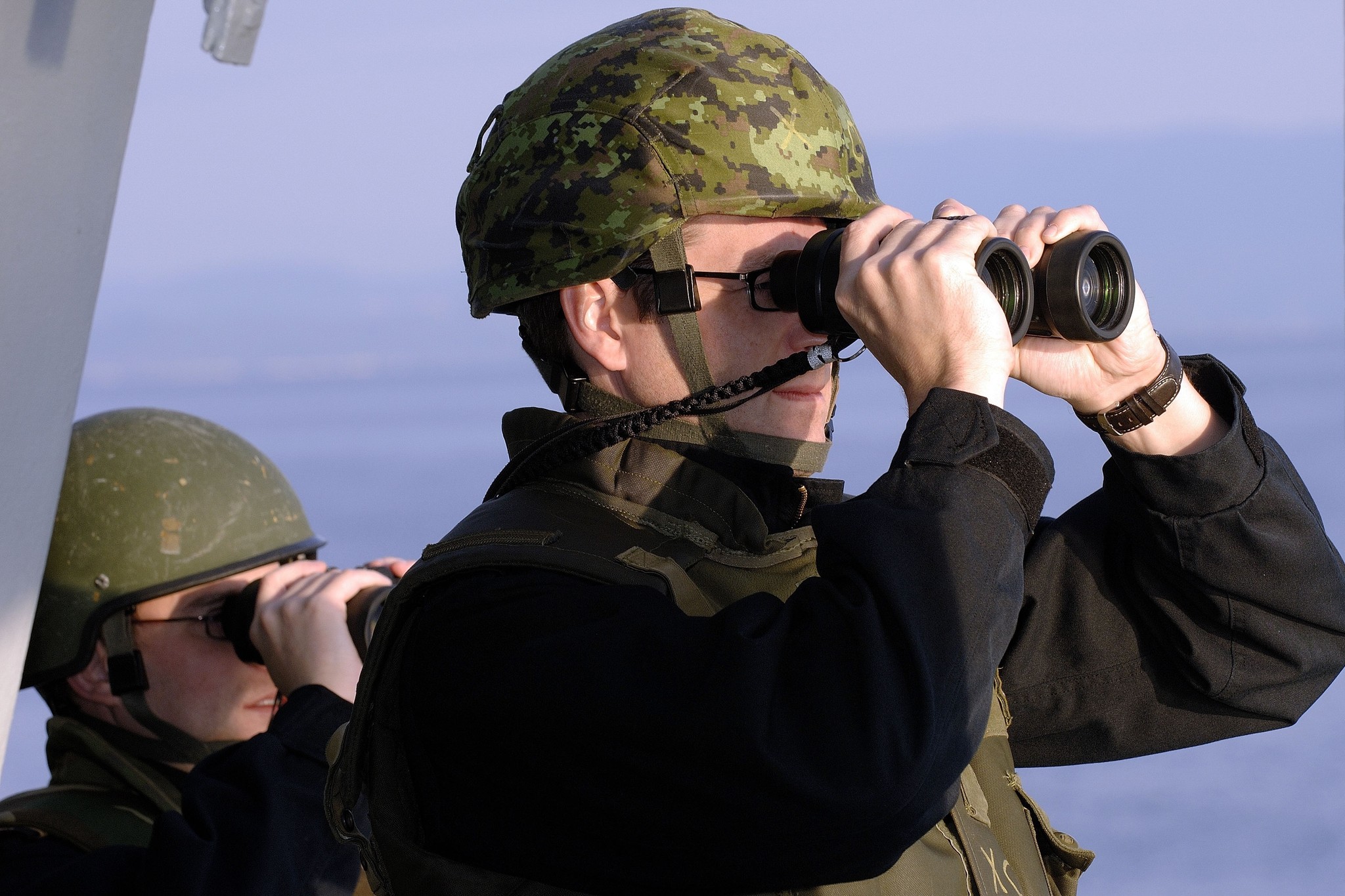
[0,403,413,896]
[324,0,1345,896]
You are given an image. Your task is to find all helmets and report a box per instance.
[455,5,883,321]
[20,405,326,691]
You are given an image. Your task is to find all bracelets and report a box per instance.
[1074,331,1185,439]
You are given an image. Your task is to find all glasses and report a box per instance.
[128,602,233,640]
[612,263,789,313]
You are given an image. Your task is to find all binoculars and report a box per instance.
[768,224,1137,349]
[227,578,400,666]
